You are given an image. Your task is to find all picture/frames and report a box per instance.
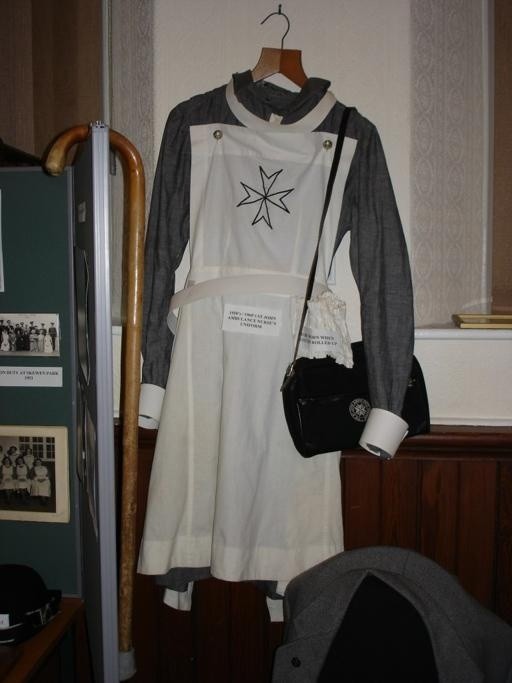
[449,313,512,331]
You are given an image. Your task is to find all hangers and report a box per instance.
[251,12,310,88]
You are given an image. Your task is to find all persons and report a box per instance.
[0,444,52,506]
[0,318,57,353]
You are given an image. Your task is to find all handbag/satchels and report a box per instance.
[282,341,429,458]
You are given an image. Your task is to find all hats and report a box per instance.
[0,565,62,644]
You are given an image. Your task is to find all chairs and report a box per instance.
[279,547,483,682]
